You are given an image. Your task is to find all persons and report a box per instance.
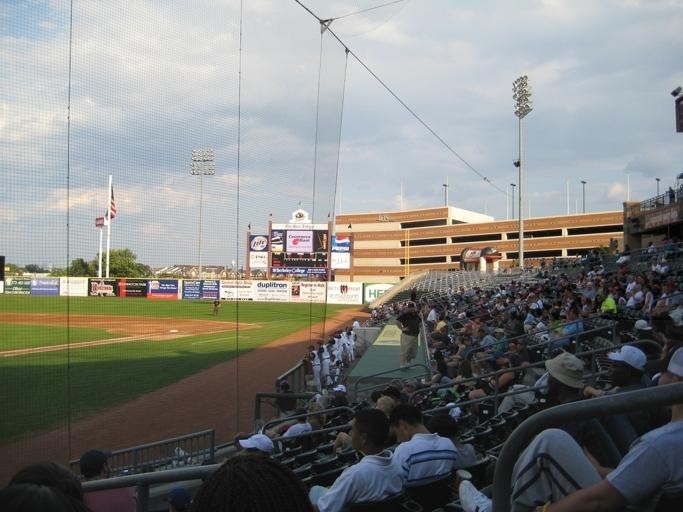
[213,298,221,316]
[669,187,675,204]
[370,235,683,371]
[2,316,683,512]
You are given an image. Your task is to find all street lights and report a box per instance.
[511,74,532,271]
[187,147,215,277]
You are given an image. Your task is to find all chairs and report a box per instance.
[136,243,682,512]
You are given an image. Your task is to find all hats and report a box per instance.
[636,319,653,331]
[372,386,400,403]
[79,450,112,476]
[607,346,648,371]
[646,344,683,377]
[495,328,505,333]
[239,432,275,453]
[546,352,584,389]
[332,385,346,393]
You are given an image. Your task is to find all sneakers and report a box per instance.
[172,446,191,467]
[459,479,493,512]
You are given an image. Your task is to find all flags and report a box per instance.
[104,181,116,221]
